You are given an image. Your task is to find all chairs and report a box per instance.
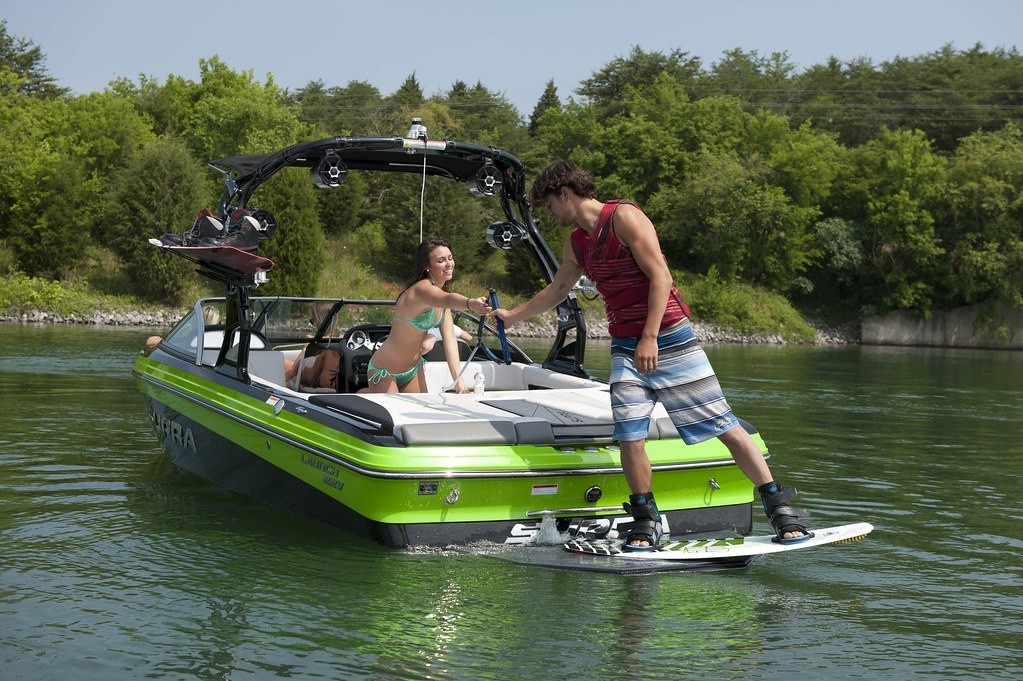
[247,350,287,388]
[295,339,341,393]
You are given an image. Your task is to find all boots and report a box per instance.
[200,209,260,254]
[759,481,810,543]
[622,492,664,552]
[161,208,225,246]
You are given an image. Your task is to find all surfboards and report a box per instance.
[148,237,275,282]
[562,521,877,561]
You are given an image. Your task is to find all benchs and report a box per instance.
[426,359,599,392]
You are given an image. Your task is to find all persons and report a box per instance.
[488,161,814,549]
[421,322,474,355]
[367,238,492,394]
[146,306,221,348]
[284,301,340,389]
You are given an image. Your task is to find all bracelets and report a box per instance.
[467,298,472,312]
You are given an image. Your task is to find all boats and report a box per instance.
[130,114,876,572]
